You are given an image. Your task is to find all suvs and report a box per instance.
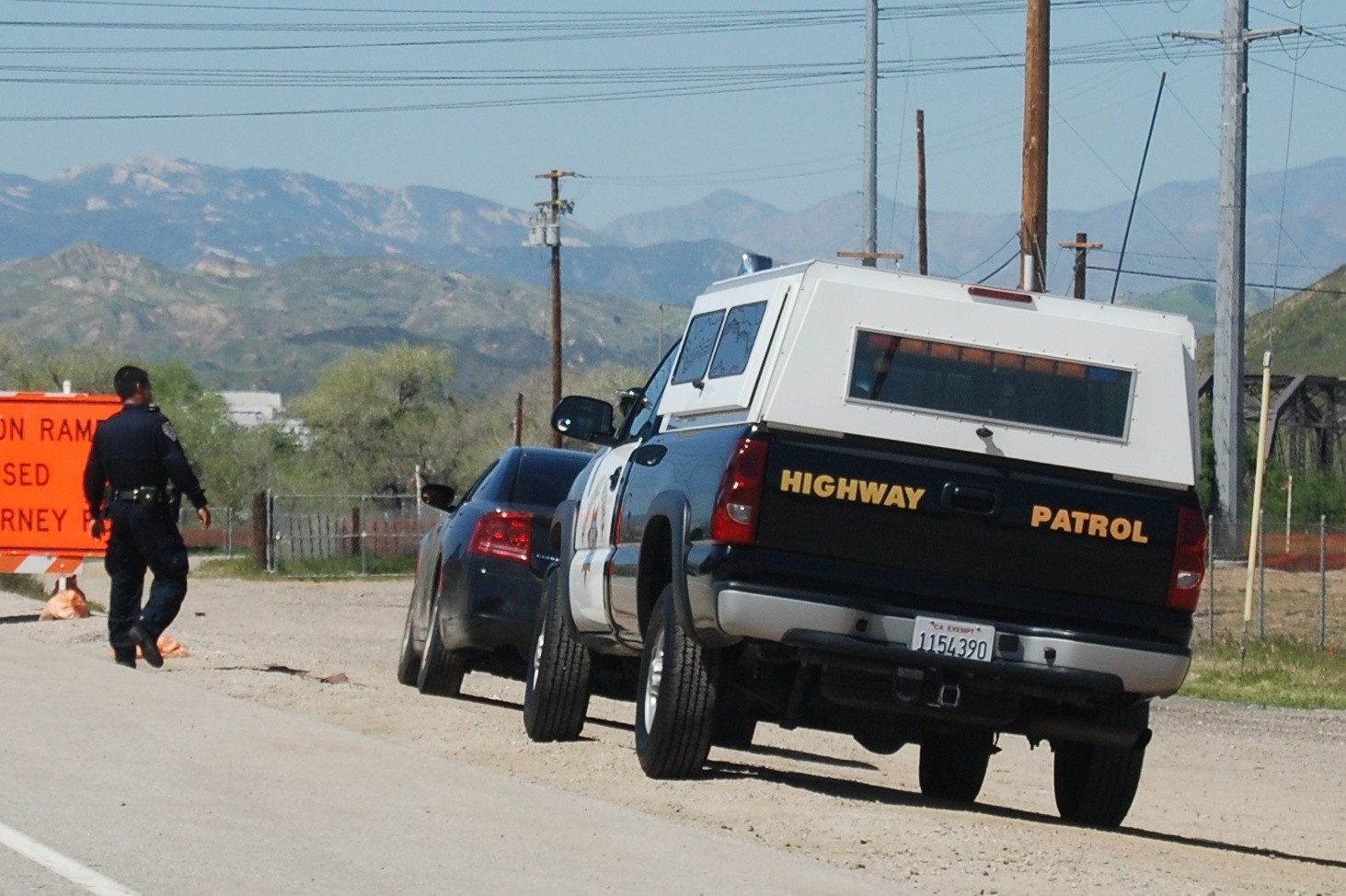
[524,258,1211,829]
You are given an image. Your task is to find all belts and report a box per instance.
[110,491,135,501]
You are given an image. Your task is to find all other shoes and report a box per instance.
[114,647,137,669]
[128,615,163,667]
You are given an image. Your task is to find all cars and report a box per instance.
[394,445,598,698]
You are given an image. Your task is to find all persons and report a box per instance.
[83,365,211,669]
